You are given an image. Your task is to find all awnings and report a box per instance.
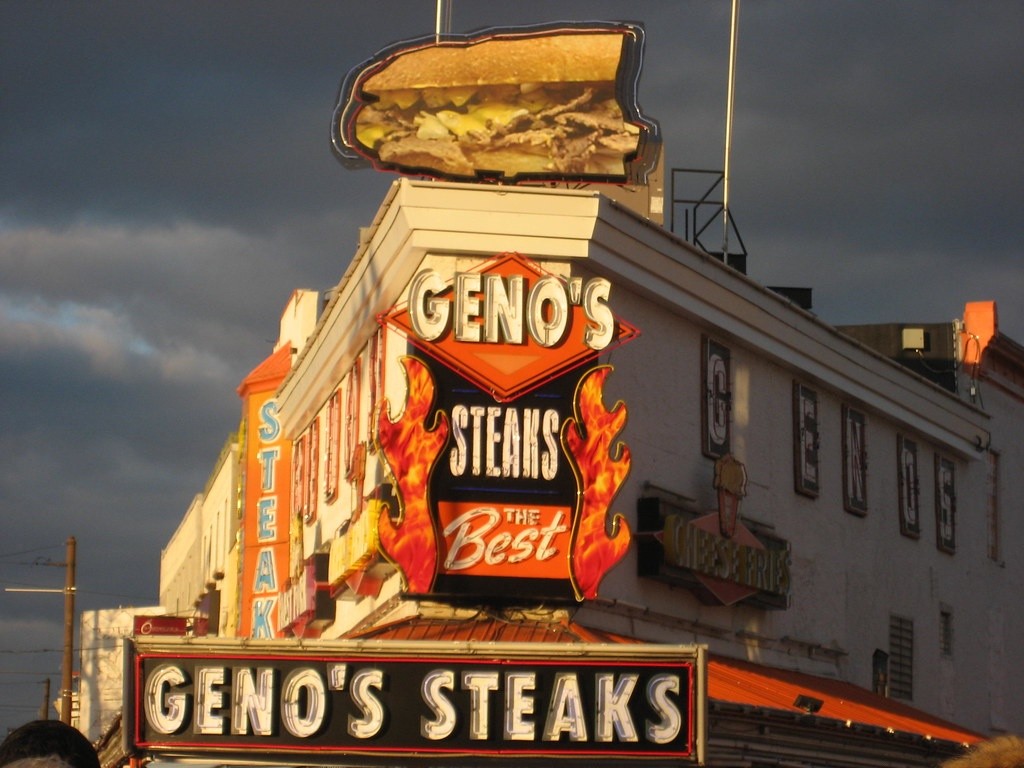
[571,623,993,748]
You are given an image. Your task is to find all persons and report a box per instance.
[1,720,100,768]
